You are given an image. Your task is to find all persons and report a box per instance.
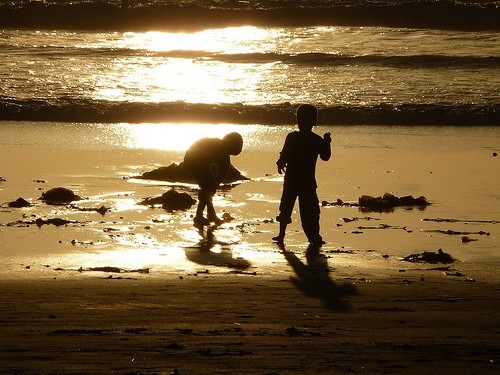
[272,104,331,245]
[184,132,243,226]
